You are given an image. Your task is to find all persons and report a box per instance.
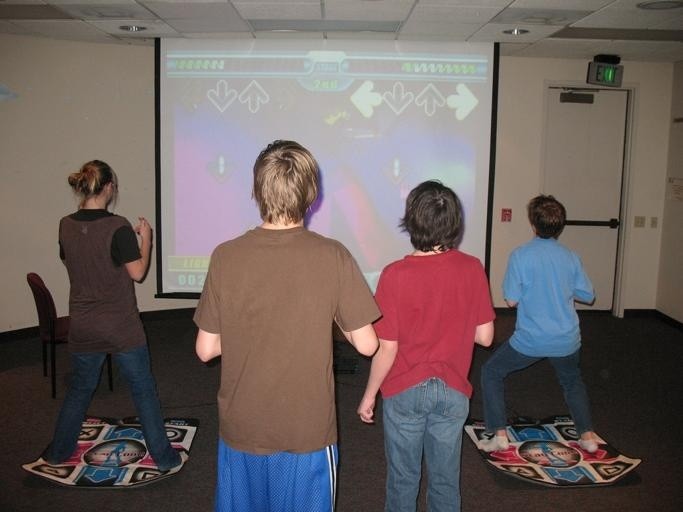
[192,139,383,512]
[356,179,496,512]
[476,194,599,453]
[43,160,191,472]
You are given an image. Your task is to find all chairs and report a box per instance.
[24,271,114,399]
